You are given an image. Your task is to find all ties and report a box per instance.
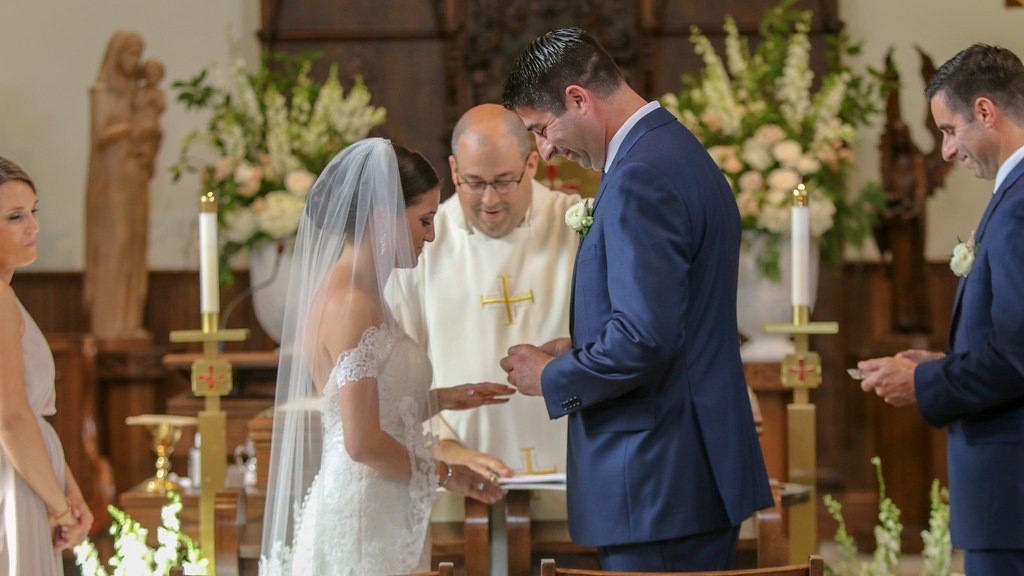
[601,168,606,179]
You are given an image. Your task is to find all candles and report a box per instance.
[199,193,220,313]
[791,187,809,306]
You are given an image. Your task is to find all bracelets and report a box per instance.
[51,498,72,523]
[437,460,453,489]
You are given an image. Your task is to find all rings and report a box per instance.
[478,483,485,491]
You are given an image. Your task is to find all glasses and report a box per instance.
[453,154,530,194]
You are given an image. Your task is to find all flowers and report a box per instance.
[830,461,952,576]
[946,230,980,277]
[73,493,205,576]
[171,47,386,255]
[564,197,594,235]
[648,0,878,239]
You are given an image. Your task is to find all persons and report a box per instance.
[85,30,167,340]
[0,154,94,576]
[259,139,517,576]
[500,26,776,573]
[382,103,601,522]
[858,44,1024,576]
[873,120,936,334]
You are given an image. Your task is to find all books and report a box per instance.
[492,474,567,493]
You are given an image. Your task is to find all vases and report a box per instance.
[735,229,819,363]
[248,237,298,345]
